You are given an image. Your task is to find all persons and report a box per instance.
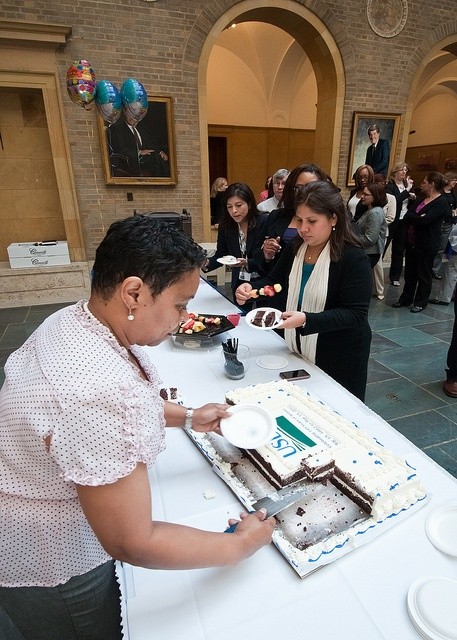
[255,163,331,276]
[268,177,273,198]
[0,212,278,640]
[383,160,417,288]
[430,171,456,280]
[197,181,270,317]
[258,175,272,204]
[213,208,227,229]
[255,168,291,213]
[354,183,388,302]
[425,220,456,307]
[209,177,229,226]
[363,123,389,181]
[106,114,168,178]
[234,179,372,403]
[391,171,456,314]
[445,308,456,401]
[344,164,374,221]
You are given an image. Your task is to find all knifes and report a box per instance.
[222,475,322,533]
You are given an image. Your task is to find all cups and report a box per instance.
[228,314,240,326]
[222,343,252,380]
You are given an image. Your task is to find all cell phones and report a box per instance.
[280,369,312,382]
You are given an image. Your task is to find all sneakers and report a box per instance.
[432,273,442,280]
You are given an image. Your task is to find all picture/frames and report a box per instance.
[346,111,401,190]
[97,93,178,186]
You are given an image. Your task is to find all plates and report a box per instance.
[255,354,289,370]
[219,403,279,451]
[216,257,241,266]
[170,314,235,339]
[425,504,457,557]
[406,575,457,640]
[245,307,285,331]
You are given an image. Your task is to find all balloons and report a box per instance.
[94,78,123,127]
[119,77,148,128]
[64,57,96,110]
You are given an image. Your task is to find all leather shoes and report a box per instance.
[410,305,424,312]
[392,302,400,307]
[443,379,457,397]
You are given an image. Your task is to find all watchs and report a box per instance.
[183,406,194,432]
[300,320,307,330]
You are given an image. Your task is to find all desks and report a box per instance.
[111,366,456,638]
[127,276,322,386]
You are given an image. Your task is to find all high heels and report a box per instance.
[376,294,384,300]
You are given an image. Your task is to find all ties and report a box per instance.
[372,144,375,154]
[133,128,141,146]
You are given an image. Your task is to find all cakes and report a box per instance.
[254,311,275,327]
[222,378,425,521]
[301,450,335,479]
[222,256,236,261]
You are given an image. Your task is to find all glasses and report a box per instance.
[362,192,372,196]
